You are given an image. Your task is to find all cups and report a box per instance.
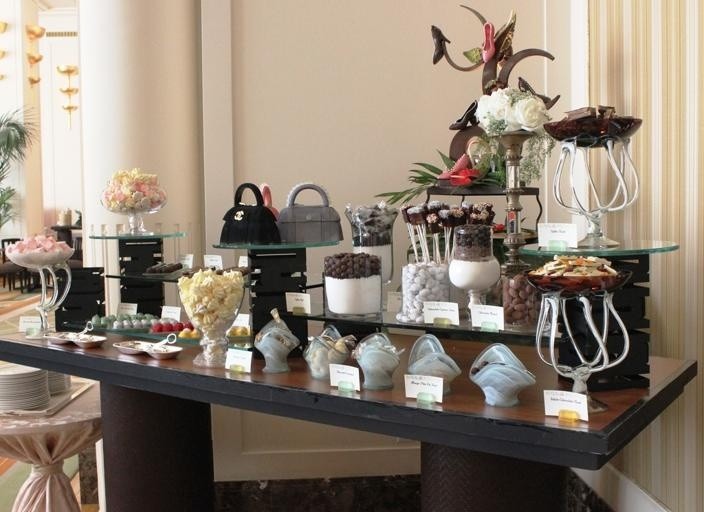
[90,223,124,236]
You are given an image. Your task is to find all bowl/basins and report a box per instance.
[524,265,633,298]
[541,118,641,147]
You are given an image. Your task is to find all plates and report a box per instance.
[43,330,106,349]
[0,362,50,415]
[111,339,183,359]
[47,370,72,396]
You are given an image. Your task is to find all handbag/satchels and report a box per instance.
[219,183,281,243]
[279,184,343,242]
[260,184,280,225]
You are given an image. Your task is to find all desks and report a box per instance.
[1,385,104,512]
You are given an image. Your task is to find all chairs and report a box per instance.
[1,239,51,292]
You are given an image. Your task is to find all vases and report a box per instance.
[475,86,548,134]
[483,135,537,265]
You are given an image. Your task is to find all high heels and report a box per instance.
[431,25,450,64]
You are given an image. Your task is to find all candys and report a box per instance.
[470,360,506,374]
[324,201,622,325]
[102,167,165,210]
[4,234,72,254]
[90,311,247,338]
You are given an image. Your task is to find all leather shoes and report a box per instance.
[436,153,469,180]
[448,99,476,130]
[481,22,495,64]
[518,78,551,103]
